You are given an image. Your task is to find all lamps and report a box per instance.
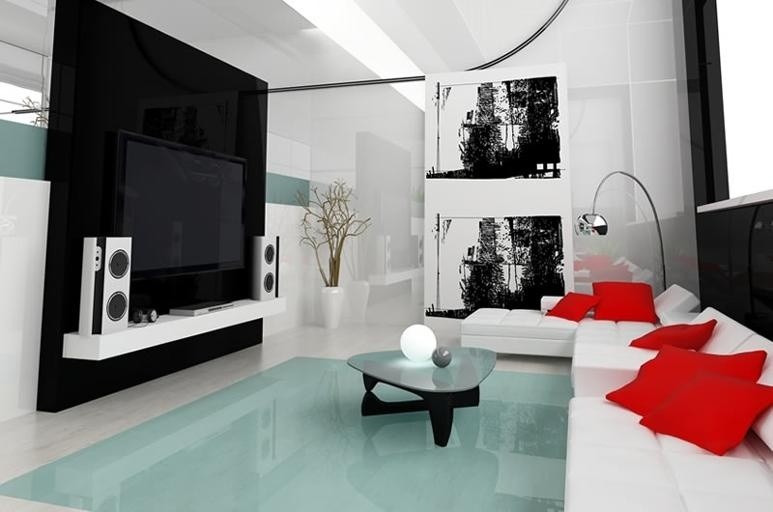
[574,169,669,290]
[401,323,436,363]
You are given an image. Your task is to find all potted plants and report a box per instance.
[342,230,370,321]
[295,176,368,327]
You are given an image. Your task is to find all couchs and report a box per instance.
[456,278,773,511]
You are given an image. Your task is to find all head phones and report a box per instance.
[132,307,158,323]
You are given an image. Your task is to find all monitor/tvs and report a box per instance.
[113,128,247,277]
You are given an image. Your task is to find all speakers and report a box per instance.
[249,233,282,301]
[76,237,132,335]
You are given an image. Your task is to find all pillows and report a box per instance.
[604,343,763,417]
[571,252,634,284]
[630,319,717,350]
[544,288,596,322]
[592,283,658,324]
[640,365,773,455]
